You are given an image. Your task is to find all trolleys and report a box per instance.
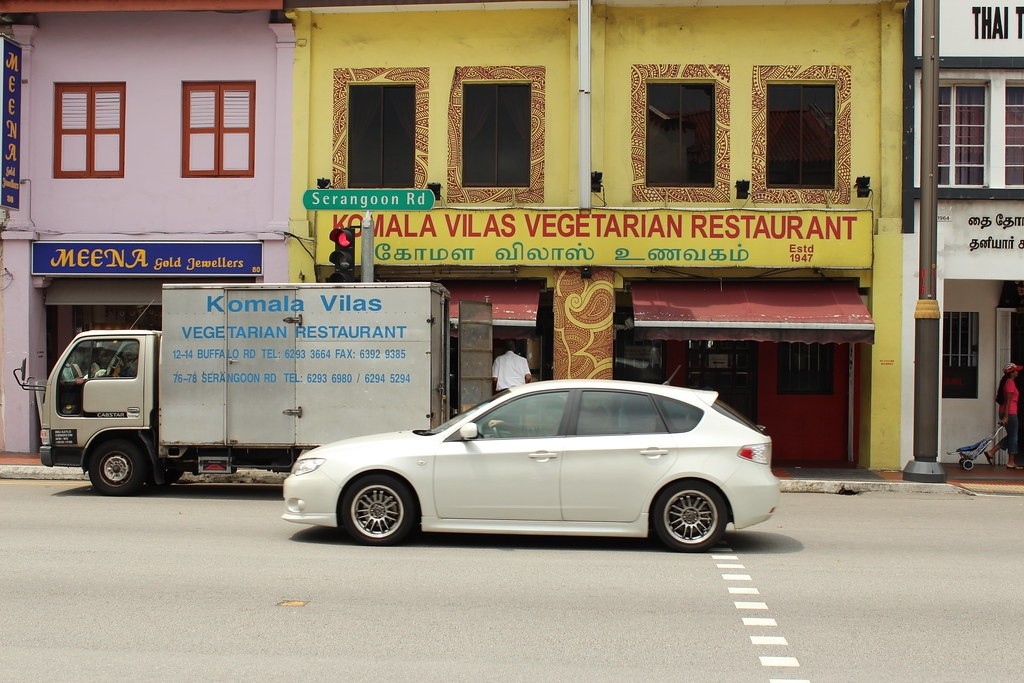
[946,424,1004,470]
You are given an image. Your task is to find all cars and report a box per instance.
[281,378,779,547]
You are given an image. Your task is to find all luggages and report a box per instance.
[946,425,1003,470]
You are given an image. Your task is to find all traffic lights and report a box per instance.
[327,226,356,283]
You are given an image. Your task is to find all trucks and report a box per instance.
[10,281,496,497]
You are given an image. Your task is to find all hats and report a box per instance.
[1003,363,1023,374]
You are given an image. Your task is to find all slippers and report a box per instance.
[984,451,995,466]
[1006,463,1024,470]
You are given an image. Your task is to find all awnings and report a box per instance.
[629,281,876,346]
[440,278,541,328]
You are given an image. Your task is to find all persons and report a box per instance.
[489,401,541,436]
[984,363,1023,469]
[492,339,531,395]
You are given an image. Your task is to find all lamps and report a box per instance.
[582,265,592,280]
[591,171,608,206]
[428,183,442,201]
[736,179,755,207]
[316,178,335,190]
[853,175,875,208]
